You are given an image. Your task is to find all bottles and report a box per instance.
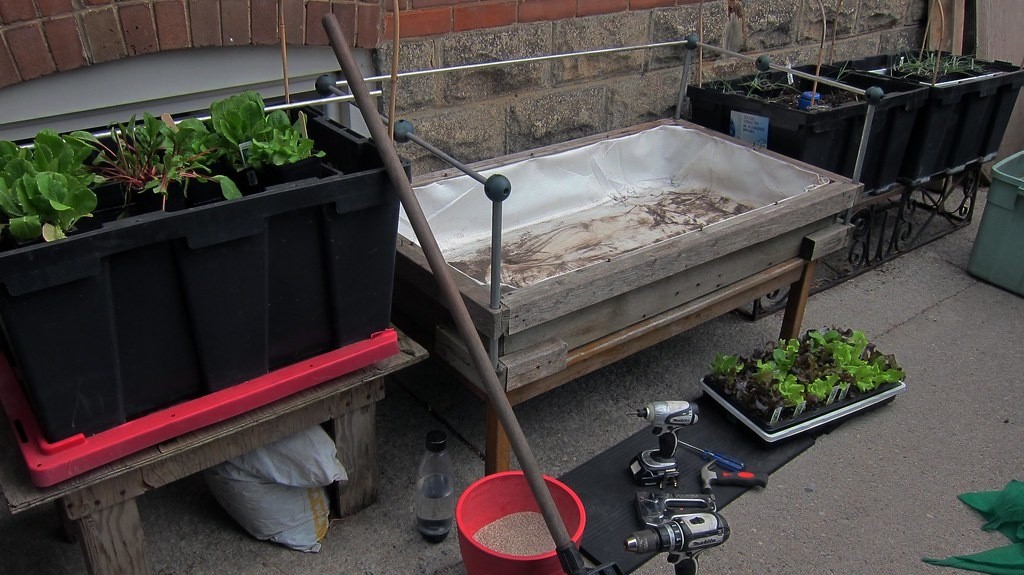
[416,431,455,544]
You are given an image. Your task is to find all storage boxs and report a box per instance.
[967,151,1024,295]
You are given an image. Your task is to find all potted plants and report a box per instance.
[701,327,908,448]
[1,94,412,474]
[688,64,930,197]
[824,50,1024,188]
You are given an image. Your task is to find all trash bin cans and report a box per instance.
[967,149,1024,298]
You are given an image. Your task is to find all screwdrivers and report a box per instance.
[677,440,745,472]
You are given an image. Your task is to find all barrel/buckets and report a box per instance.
[455,471,587,575]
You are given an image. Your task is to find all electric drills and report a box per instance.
[626,400,700,487]
[623,512,730,575]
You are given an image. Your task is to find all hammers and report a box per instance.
[700,459,769,494]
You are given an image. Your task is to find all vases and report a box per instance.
[455,471,586,575]
